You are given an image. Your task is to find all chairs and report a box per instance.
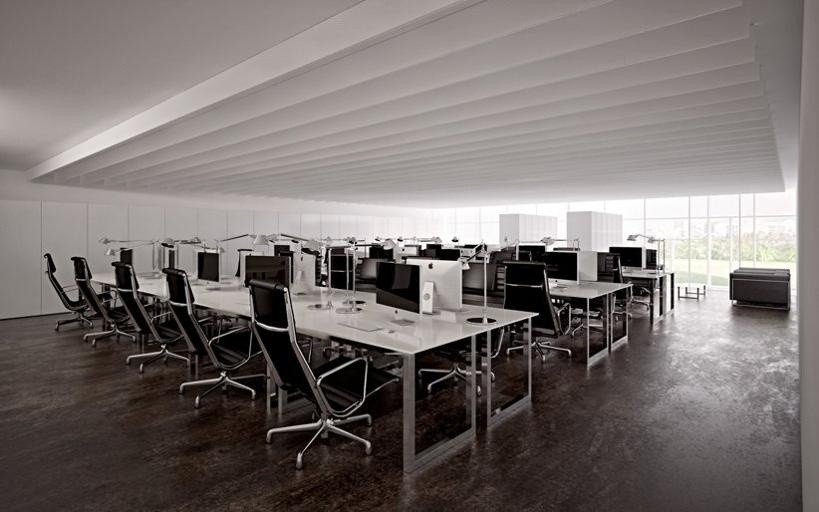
[42,230,679,474]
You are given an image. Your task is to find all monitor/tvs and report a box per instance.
[119,243,659,297]
[406,259,462,316]
[375,261,423,327]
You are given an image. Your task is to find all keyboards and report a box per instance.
[340,317,384,332]
[235,298,250,305]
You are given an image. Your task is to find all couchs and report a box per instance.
[725,266,792,313]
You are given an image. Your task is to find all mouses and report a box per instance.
[315,304,321,308]
